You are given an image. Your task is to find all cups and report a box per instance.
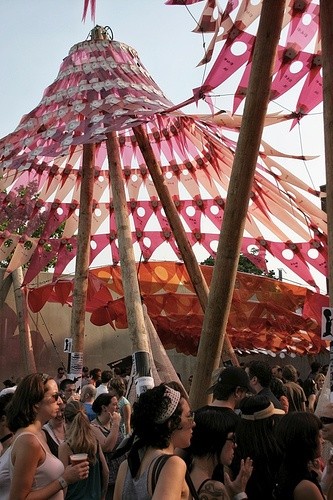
[69,453,88,476]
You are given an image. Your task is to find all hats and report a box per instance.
[219,367,257,394]
[240,400,286,421]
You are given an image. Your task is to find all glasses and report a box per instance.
[107,386,114,392]
[226,432,237,445]
[64,388,77,392]
[177,411,196,423]
[44,392,63,402]
[59,372,64,375]
[320,416,333,425]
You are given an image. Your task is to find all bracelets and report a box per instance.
[233,492,248,500]
[58,477,67,489]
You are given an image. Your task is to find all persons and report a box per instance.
[0,362,333,500]
